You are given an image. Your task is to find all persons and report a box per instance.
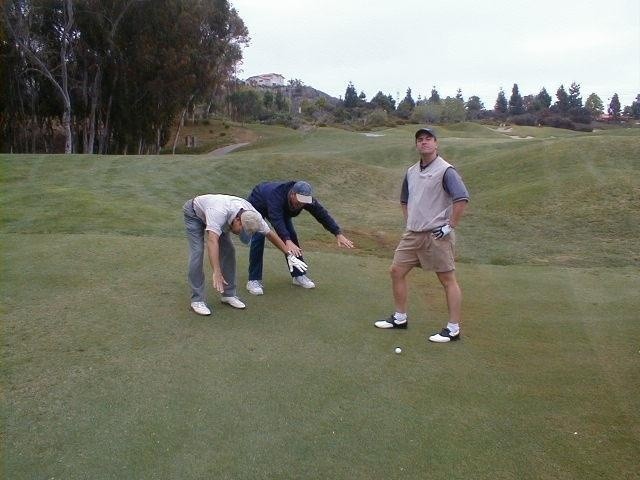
[182,194,308,316]
[247,180,355,296]
[374,128,470,343]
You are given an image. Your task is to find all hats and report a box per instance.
[415,128,437,139]
[239,210,260,245]
[292,181,313,204]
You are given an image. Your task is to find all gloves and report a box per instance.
[431,224,454,240]
[285,251,308,272]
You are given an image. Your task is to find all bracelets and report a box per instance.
[285,251,292,256]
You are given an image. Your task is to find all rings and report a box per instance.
[347,241,350,243]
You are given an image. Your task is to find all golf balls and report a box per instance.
[395,347,401,354]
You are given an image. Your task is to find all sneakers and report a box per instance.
[246,280,264,296]
[374,314,408,329]
[220,295,245,309]
[291,274,316,289]
[429,327,461,343]
[191,301,211,316]
